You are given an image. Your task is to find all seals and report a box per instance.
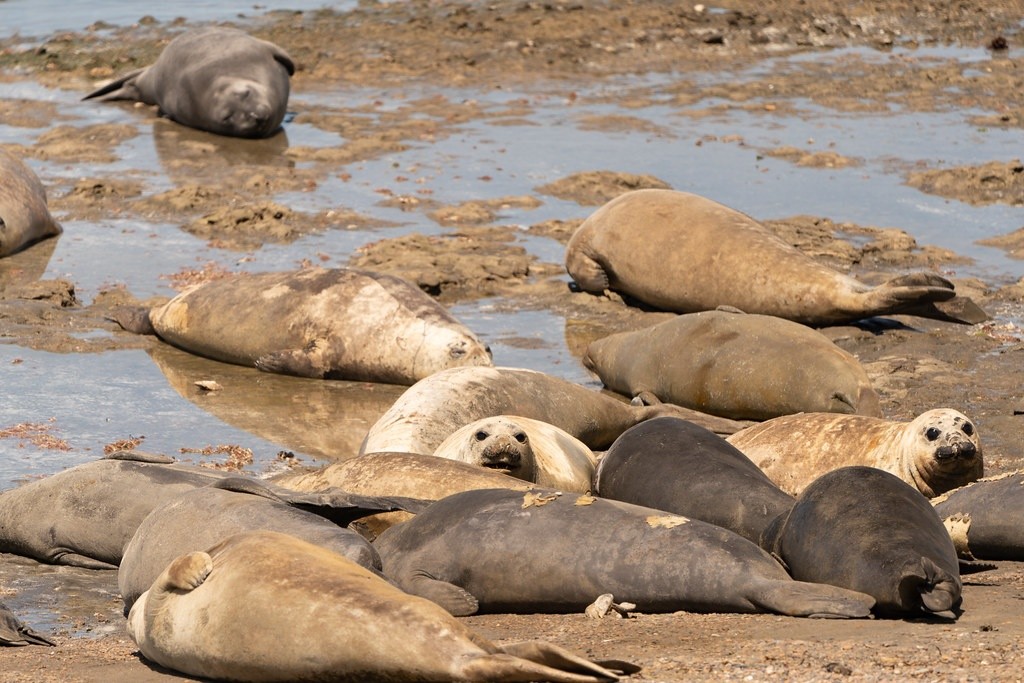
[0,148,62,257]
[81,25,295,140]
[0,188,1024,683]
